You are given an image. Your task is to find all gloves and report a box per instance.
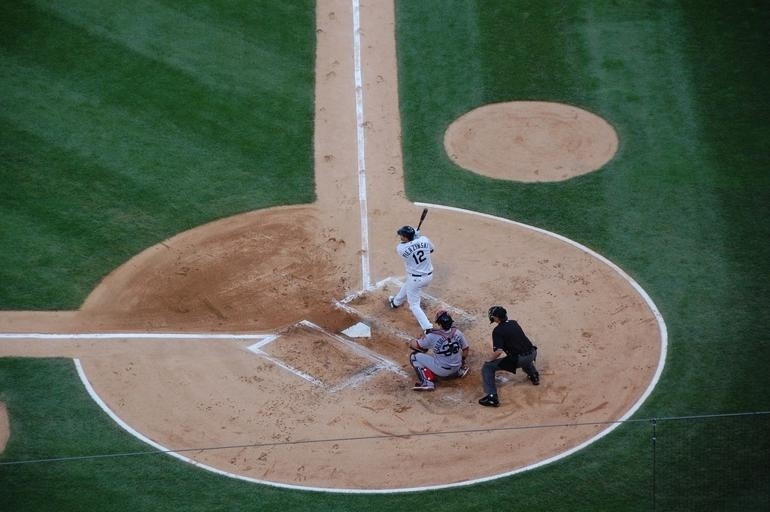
[415,227,421,236]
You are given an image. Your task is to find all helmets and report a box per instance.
[488,306,508,324]
[433,310,454,331]
[396,225,415,239]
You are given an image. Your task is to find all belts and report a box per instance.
[412,272,432,277]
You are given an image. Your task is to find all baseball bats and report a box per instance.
[416,209,429,232]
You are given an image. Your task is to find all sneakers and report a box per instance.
[527,371,539,385]
[460,364,469,378]
[479,396,499,407]
[410,384,434,390]
[389,295,397,309]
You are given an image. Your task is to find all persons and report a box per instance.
[406,309,470,391]
[477,305,541,407]
[388,225,436,335]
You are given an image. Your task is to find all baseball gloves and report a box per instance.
[405,338,430,353]
